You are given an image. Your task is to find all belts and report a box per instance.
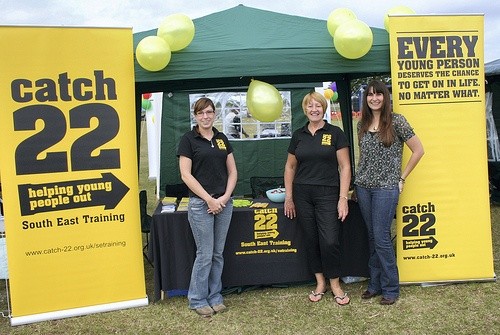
[207,193,223,199]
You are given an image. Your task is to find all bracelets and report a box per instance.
[340,196,348,200]
[399,176,406,183]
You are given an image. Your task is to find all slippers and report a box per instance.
[308,288,328,302]
[331,290,350,305]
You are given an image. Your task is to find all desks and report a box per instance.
[148,200,371,303]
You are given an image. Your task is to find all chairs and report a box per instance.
[250,176,285,200]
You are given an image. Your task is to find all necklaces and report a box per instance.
[373,126,377,130]
[211,140,214,148]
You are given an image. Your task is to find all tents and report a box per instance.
[134,4,392,176]
[484,59,500,205]
[146,87,331,178]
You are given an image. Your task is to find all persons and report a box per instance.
[223,104,249,139]
[283,92,351,305]
[176,97,238,316]
[355,79,425,306]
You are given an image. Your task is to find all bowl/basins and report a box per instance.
[266,188,286,202]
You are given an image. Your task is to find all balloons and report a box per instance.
[384,6,416,32]
[157,13,195,51]
[333,20,373,59]
[136,35,172,72]
[246,78,282,122]
[142,93,152,110]
[327,7,357,37]
[324,82,338,101]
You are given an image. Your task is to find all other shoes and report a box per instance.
[212,303,227,312]
[196,305,214,316]
[380,297,395,304]
[362,289,376,299]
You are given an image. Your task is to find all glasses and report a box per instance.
[196,110,216,117]
